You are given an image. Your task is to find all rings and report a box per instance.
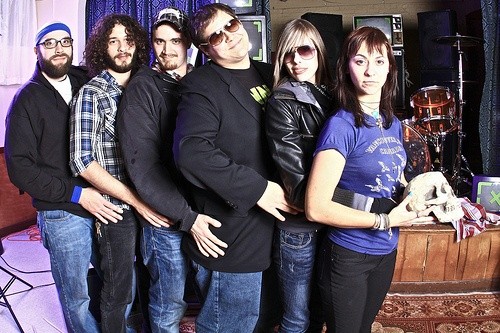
[412,225,413,227]
[417,213,418,218]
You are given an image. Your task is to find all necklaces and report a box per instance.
[362,103,380,117]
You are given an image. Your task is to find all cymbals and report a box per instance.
[432,80,477,84]
[431,36,486,47]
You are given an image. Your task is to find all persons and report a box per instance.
[172,3,304,333]
[266,18,342,333]
[304,26,436,333]
[68,13,174,333]
[116,7,228,333]
[3,20,124,333]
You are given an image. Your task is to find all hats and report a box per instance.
[34,21,71,46]
[151,7,194,49]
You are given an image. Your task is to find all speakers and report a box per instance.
[301,12,344,85]
[389,49,406,110]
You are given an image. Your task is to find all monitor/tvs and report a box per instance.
[201,0,268,65]
[354,14,404,47]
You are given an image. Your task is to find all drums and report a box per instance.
[400,122,431,183]
[409,84,460,137]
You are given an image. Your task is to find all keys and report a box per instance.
[95,222,101,234]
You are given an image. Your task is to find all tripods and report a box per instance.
[445,40,475,197]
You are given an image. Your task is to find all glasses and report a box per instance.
[200,18,241,47]
[283,45,317,64]
[37,37,74,48]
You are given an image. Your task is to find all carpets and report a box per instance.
[181,295,500,333]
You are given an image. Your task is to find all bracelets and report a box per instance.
[372,213,390,231]
